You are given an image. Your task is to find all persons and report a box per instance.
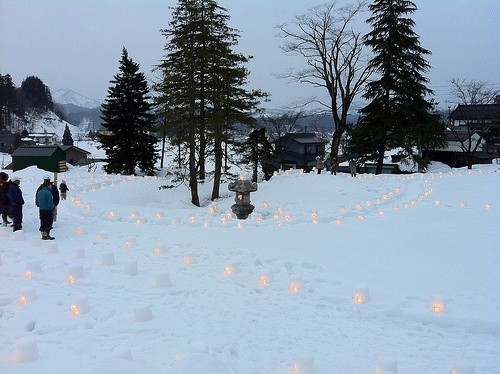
[50,182,60,221]
[36,179,54,229]
[331,156,339,175]
[37,182,55,240]
[59,180,70,200]
[7,176,24,232]
[0,172,12,224]
[349,157,356,177]
[317,153,324,174]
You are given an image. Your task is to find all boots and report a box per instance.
[44,227,55,240]
[40,227,47,240]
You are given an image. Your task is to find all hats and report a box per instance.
[11,176,21,182]
